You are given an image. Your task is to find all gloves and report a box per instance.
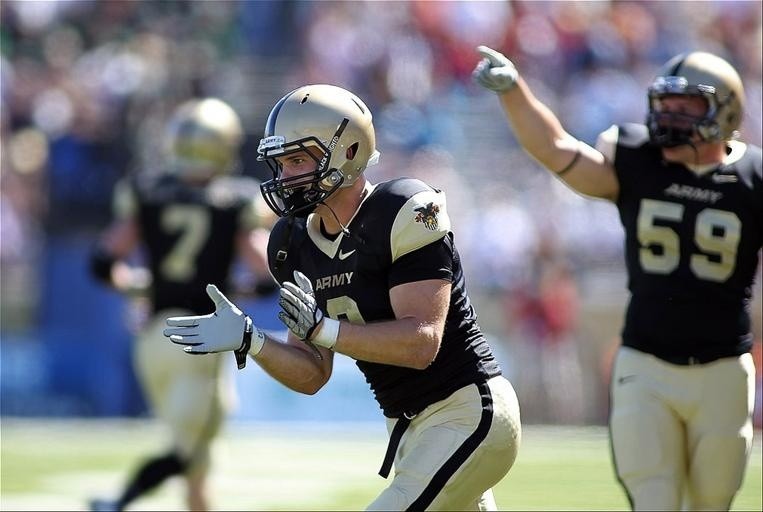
[472,46,519,93]
[280,270,340,360]
[163,284,265,370]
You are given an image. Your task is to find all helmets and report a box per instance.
[167,97,242,178]
[647,52,744,150]
[257,84,380,216]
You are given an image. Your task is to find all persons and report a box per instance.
[162,85,519,511]
[87,99,278,511]
[475,46,763,511]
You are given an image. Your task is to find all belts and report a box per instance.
[379,402,431,479]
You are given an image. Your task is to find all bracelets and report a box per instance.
[312,317,340,349]
